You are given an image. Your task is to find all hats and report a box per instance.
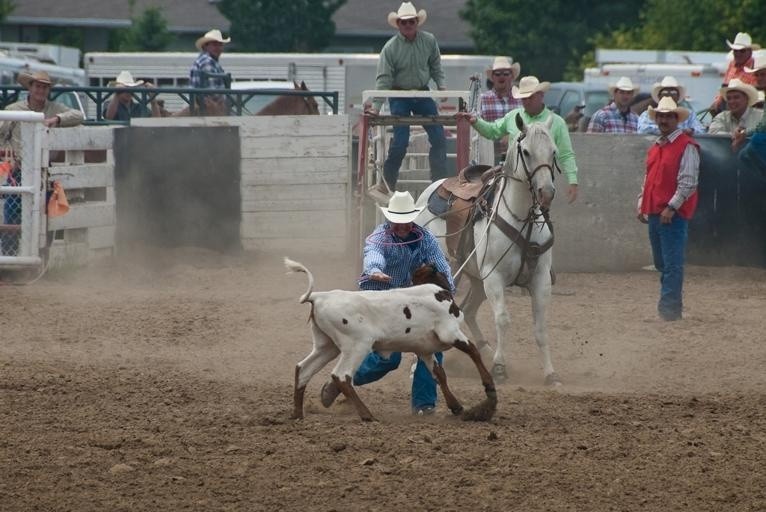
[195,29,231,52]
[107,69,144,88]
[17,70,54,89]
[485,56,521,82]
[647,76,691,124]
[379,191,428,226]
[610,75,639,103]
[720,32,766,107]
[511,76,551,99]
[387,2,427,29]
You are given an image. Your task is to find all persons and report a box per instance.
[188,30,238,116]
[586,76,641,134]
[459,75,585,203]
[708,31,766,116]
[742,49,766,111]
[702,78,766,151]
[320,189,458,421]
[2,71,84,259]
[369,0,448,196]
[636,75,705,137]
[634,97,702,317]
[477,56,523,163]
[103,72,160,120]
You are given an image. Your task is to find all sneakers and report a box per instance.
[321,377,341,408]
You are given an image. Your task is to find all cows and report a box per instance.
[284,256,498,422]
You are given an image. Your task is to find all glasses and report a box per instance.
[494,71,510,76]
[401,19,416,25]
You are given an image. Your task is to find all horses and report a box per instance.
[409,111,564,388]
[173,93,226,117]
[254,78,321,116]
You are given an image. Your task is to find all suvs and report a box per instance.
[541,82,610,117]
